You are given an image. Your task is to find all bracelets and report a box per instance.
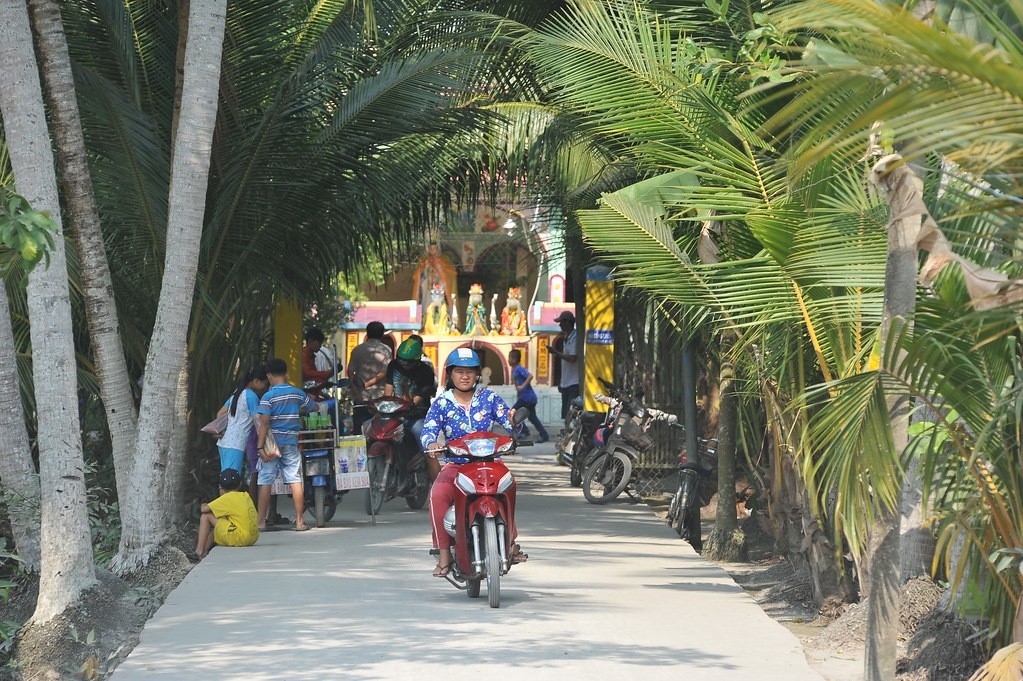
[257,447,263,450]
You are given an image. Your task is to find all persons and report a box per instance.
[544,311,578,431]
[507,349,550,444]
[257,359,319,532]
[420,347,527,577]
[194,468,259,559]
[301,327,343,394]
[349,320,441,484]
[215,364,269,493]
[419,241,527,337]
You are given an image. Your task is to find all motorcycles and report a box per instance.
[286,378,354,529]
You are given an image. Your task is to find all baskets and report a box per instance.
[620,416,654,453]
[698,443,717,468]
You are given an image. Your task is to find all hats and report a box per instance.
[219,468,241,489]
[554,310,574,322]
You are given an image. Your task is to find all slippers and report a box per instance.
[259,527,268,531]
[295,522,310,530]
[514,551,526,562]
[433,564,451,577]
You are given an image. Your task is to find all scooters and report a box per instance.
[555,394,583,466]
[351,376,437,515]
[569,376,663,506]
[423,405,534,608]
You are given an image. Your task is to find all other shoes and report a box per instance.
[267,514,289,524]
[266,518,274,524]
[536,436,548,443]
[185,551,199,560]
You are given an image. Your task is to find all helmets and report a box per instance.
[443,348,482,370]
[396,338,421,361]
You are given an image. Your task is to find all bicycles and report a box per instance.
[666,421,721,540]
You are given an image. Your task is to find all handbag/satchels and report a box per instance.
[252,411,282,458]
[201,412,228,434]
